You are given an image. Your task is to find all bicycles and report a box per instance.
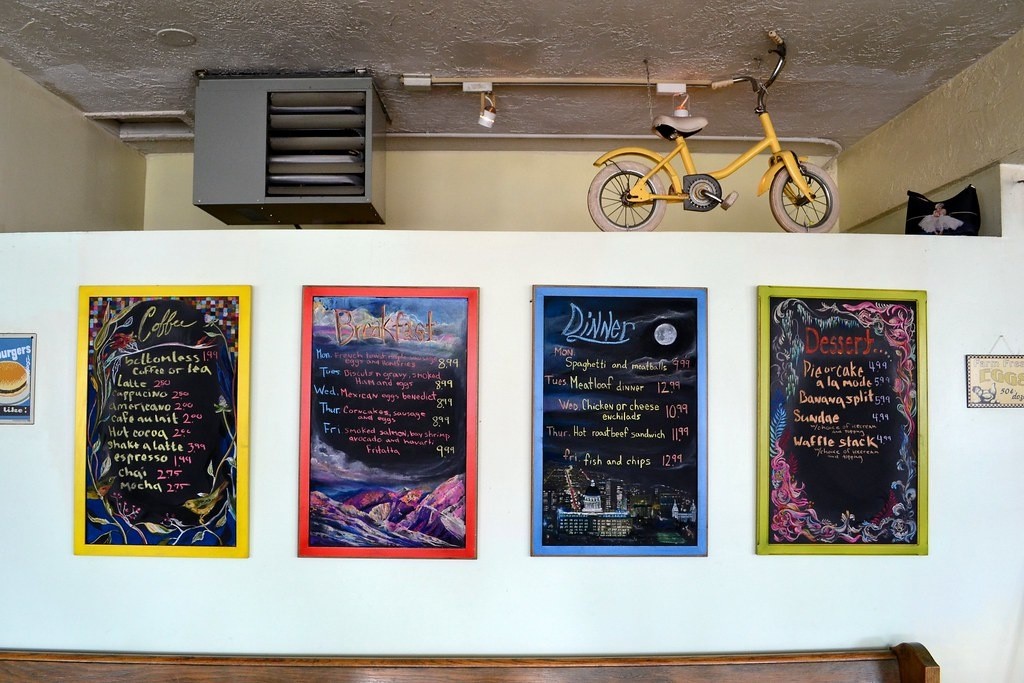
[585,28,840,234]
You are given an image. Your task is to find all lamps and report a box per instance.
[463,82,497,129]
[655,84,691,117]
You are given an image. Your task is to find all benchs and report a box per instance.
[0,643,939,683]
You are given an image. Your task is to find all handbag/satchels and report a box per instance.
[905,184,981,236]
